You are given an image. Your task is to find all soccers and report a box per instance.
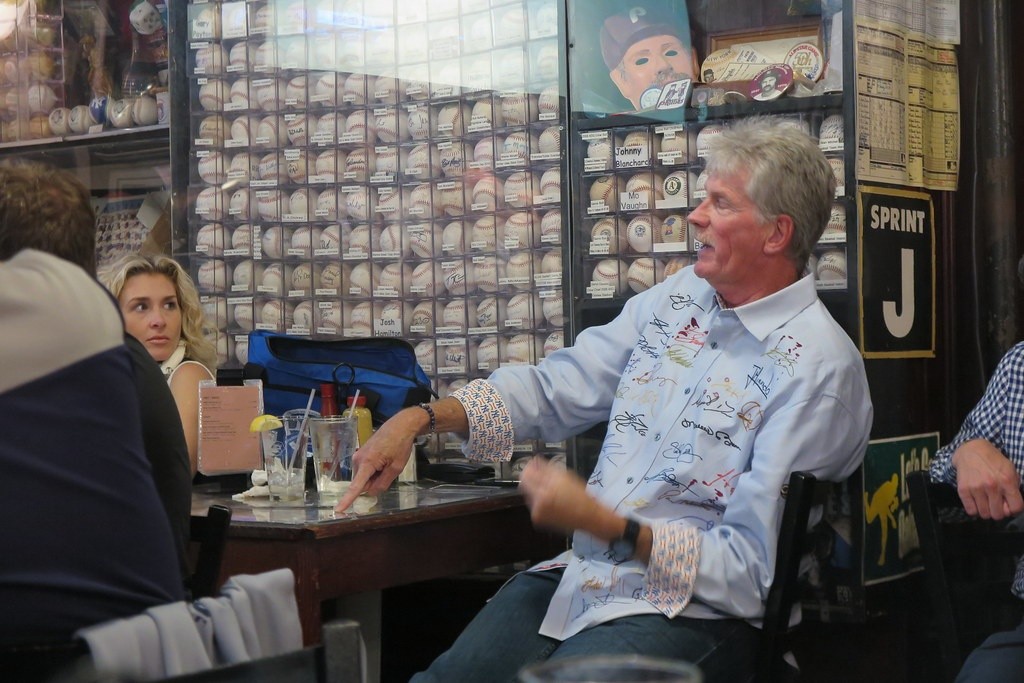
[1,0,63,135]
[68,105,91,135]
[583,116,848,295]
[108,96,132,129]
[129,96,158,125]
[187,0,565,479]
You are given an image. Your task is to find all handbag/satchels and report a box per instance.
[241,328,439,488]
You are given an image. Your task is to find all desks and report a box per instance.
[183,471,571,683]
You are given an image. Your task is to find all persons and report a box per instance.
[334,116,875,681]
[787,68,814,93]
[105,252,216,477]
[703,70,715,82]
[930,256,1024,683]
[754,69,782,100]
[0,156,193,683]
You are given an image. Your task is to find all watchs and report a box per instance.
[610,519,641,563]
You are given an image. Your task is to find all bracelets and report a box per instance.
[413,403,435,448]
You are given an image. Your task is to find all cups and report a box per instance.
[309,415,358,507]
[262,416,310,507]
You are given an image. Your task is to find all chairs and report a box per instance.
[751,470,845,683]
[905,470,1024,683]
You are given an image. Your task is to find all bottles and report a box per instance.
[319,383,340,416]
[342,396,372,450]
